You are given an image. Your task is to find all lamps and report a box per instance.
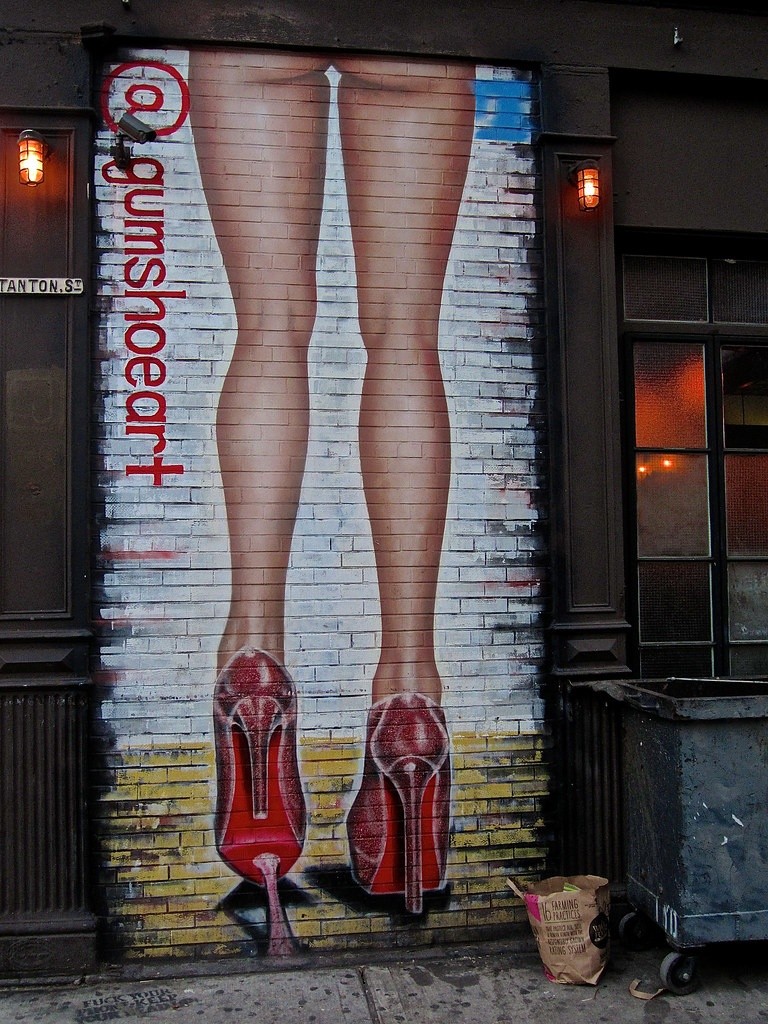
[16,129,51,187]
[576,159,600,212]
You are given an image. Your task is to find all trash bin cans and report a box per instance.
[600,672,768,994]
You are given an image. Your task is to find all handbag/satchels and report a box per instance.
[506,875,611,986]
